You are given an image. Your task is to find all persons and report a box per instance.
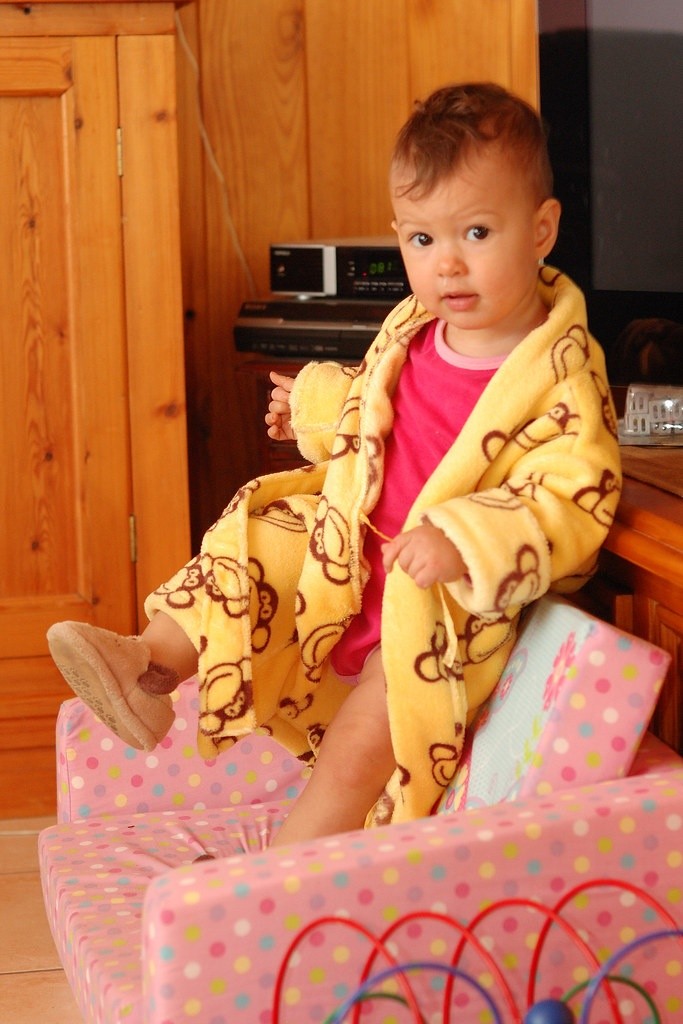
[45,83,622,848]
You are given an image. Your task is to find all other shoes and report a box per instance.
[46,621,177,752]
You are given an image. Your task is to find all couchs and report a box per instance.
[36,592,683,1024]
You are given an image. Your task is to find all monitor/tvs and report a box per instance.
[537,0,683,357]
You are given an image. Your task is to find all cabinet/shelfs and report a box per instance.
[0,0,192,820]
[600,446,682,756]
